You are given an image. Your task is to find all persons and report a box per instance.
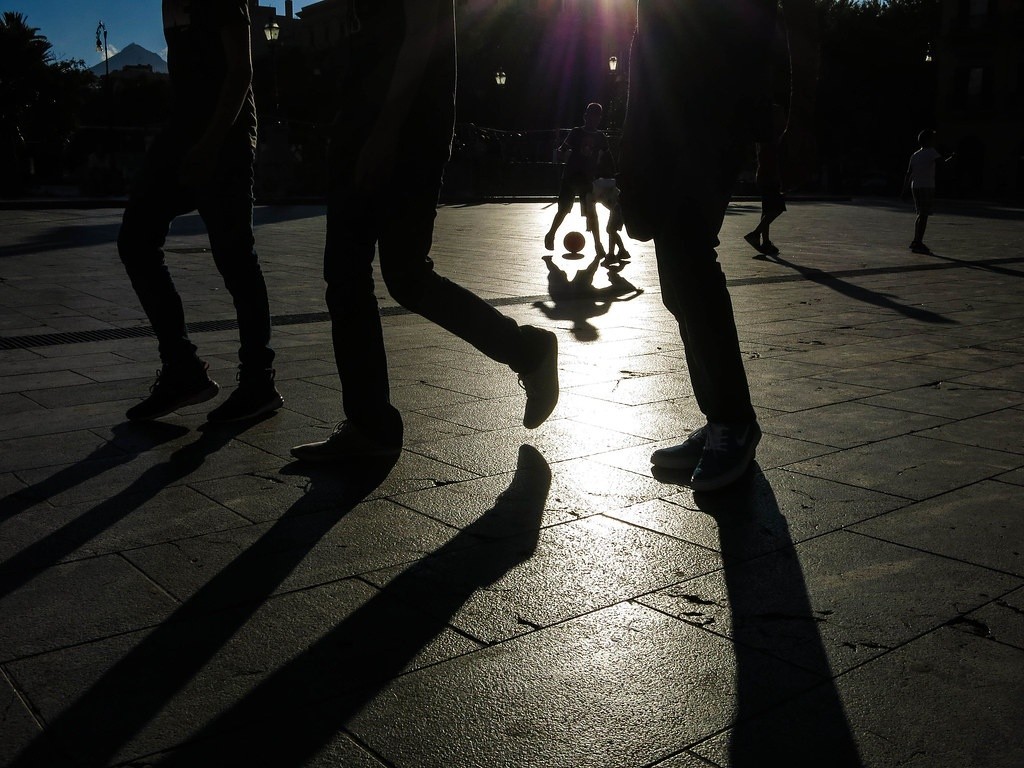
[290,0,558,462]
[899,129,948,254]
[117,0,285,426]
[545,103,630,266]
[617,0,796,494]
[744,134,787,254]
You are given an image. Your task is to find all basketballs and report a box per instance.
[563,232,585,252]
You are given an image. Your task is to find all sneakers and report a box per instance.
[691,420,762,491]
[206,373,284,424]
[125,360,220,421]
[290,418,402,461]
[652,424,707,470]
[516,328,558,429]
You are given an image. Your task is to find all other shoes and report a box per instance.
[908,241,929,254]
[602,255,617,266]
[761,243,781,254]
[745,232,760,250]
[545,234,553,249]
[597,249,605,258]
[617,252,629,259]
